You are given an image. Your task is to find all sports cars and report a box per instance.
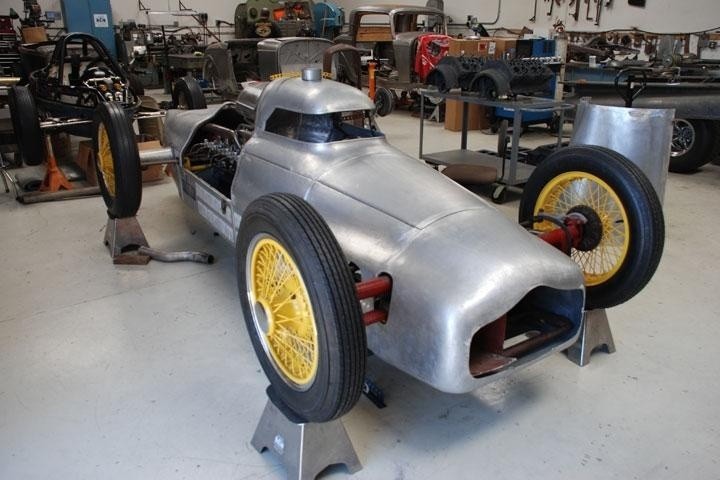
[170,35,394,129]
[89,62,664,422]
[7,31,209,165]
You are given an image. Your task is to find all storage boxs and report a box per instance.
[22,26,48,44]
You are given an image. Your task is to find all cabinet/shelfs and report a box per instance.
[414,87,574,197]
[60,0,117,59]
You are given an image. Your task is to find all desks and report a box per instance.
[161,53,204,79]
